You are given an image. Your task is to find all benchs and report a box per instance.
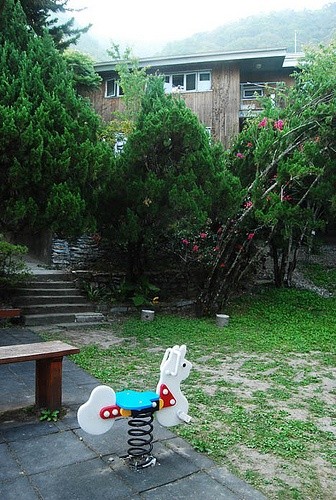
[0,339,82,419]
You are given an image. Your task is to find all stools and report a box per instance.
[76,343,194,470]
